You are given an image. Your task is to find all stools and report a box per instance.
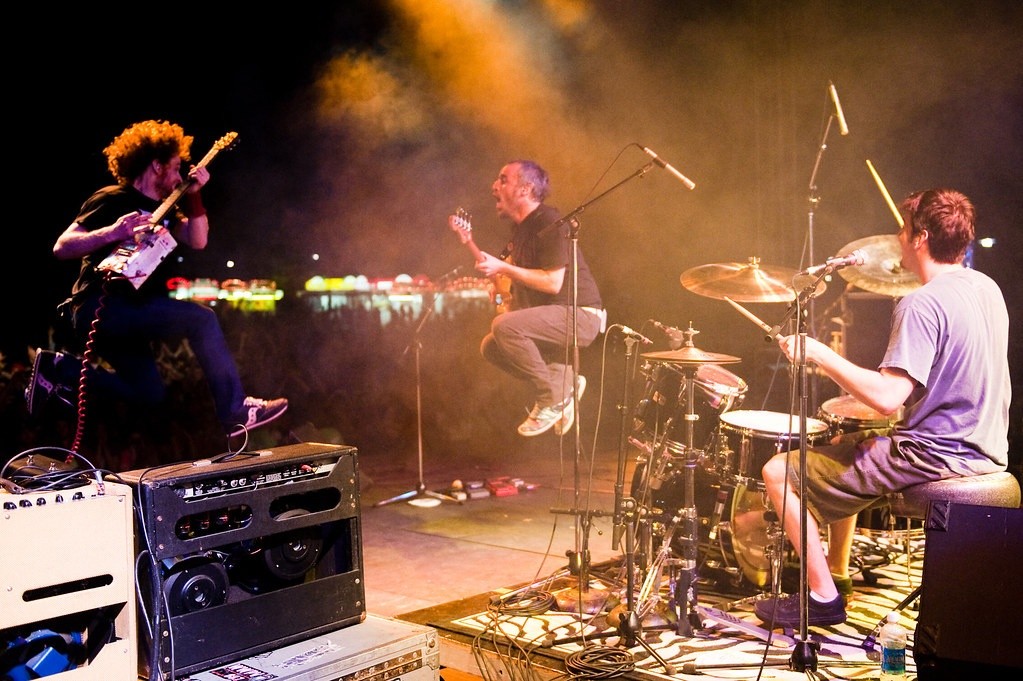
[861,473,1021,653]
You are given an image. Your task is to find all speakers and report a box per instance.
[916,500,1023,681]
[0,474,138,681]
[105,441,366,681]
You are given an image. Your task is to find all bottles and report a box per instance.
[879,611,907,681]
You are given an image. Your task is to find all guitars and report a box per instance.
[449,207,515,315]
[94,131,241,291]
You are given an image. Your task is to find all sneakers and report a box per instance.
[555,375,586,436]
[754,591,847,626]
[225,396,288,437]
[518,396,574,437]
[783,568,852,597]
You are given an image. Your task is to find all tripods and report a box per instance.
[373,270,465,508]
[488,156,916,681]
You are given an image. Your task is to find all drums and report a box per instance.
[629,453,785,589]
[715,410,830,492]
[818,394,906,440]
[632,362,749,454]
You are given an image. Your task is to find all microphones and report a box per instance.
[708,483,728,544]
[615,323,653,345]
[635,143,695,190]
[800,249,869,275]
[828,79,849,135]
[438,265,465,283]
[650,319,671,334]
[662,363,721,406]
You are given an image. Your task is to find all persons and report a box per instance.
[24,118,289,438]
[473,160,602,437]
[754,185,1012,626]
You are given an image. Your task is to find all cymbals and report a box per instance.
[679,261,827,303]
[834,234,925,296]
[639,347,742,369]
